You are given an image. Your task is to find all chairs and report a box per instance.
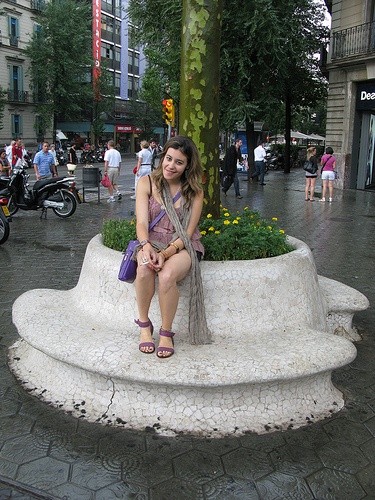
[83,167,101,204]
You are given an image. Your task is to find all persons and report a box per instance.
[305,147,320,201]
[222,139,249,198]
[0,139,30,187]
[134,135,205,358]
[33,141,58,181]
[250,140,268,185]
[103,141,122,202]
[68,143,78,177]
[318,147,336,202]
[130,140,157,199]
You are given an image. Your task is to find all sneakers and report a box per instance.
[106,197,115,202]
[130,196,135,199]
[118,193,122,200]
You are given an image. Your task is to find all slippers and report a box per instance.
[310,199,315,201]
[305,197,309,201]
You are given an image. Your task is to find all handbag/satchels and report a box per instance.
[118,240,142,283]
[304,157,317,174]
[101,175,111,187]
[223,176,231,188]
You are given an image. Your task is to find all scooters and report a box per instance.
[0,186,16,245]
[23,151,32,168]
[56,146,106,166]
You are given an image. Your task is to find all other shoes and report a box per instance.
[235,196,241,199]
[329,198,332,202]
[250,176,253,184]
[259,182,266,185]
[319,198,326,201]
[223,191,227,196]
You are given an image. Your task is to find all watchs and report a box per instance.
[140,239,150,247]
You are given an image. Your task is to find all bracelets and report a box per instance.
[168,243,179,254]
[160,249,168,261]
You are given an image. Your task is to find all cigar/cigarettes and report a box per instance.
[140,260,150,267]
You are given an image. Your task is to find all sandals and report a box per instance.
[157,329,175,357]
[134,318,156,354]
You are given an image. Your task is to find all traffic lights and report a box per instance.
[161,99,174,127]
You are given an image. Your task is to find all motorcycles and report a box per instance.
[0,162,83,222]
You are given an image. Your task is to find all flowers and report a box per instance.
[200,203,290,259]
